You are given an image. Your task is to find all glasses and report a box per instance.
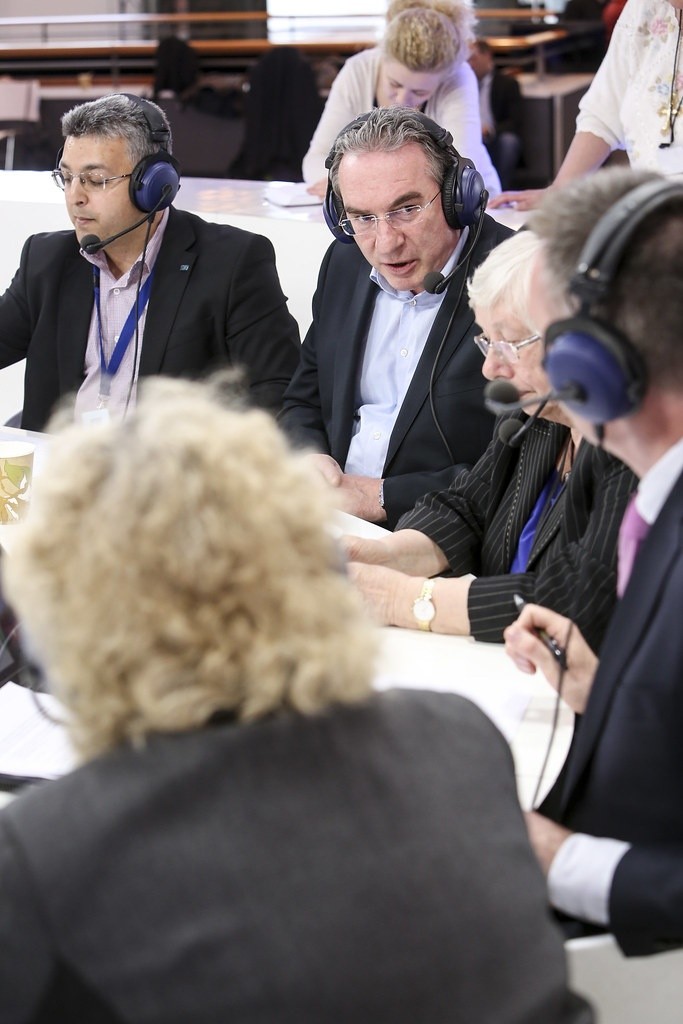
[338,189,442,236]
[51,168,132,192]
[473,332,540,364]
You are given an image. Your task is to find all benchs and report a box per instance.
[0,75,42,169]
[154,87,555,192]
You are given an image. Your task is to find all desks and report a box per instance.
[0,169,575,813]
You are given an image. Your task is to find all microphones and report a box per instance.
[80,195,164,254]
[499,391,551,446]
[484,382,573,415]
[423,208,485,294]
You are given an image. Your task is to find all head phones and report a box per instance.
[61,92,181,224]
[542,177,683,427]
[322,109,489,245]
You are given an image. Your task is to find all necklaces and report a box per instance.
[659,9,683,149]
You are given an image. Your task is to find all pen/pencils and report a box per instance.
[512,593,564,659]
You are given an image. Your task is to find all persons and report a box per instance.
[0,92,301,432]
[275,103,515,524]
[487,0,683,211]
[337,226,640,661]
[0,364,598,1024]
[303,0,502,202]
[503,169,682,958]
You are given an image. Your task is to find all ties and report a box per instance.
[616,493,653,601]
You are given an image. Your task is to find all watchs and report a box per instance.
[412,580,436,632]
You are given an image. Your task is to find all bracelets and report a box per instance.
[380,476,383,509]
